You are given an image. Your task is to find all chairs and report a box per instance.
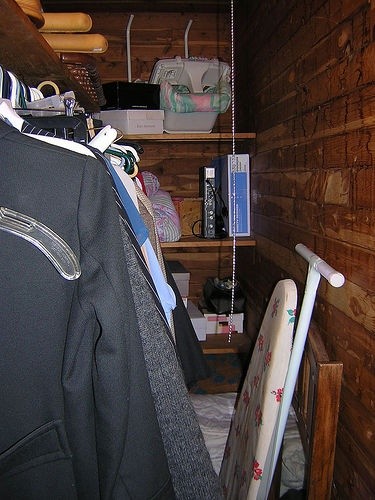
[187,315,344,500]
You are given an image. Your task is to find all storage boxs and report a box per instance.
[166,257,191,298]
[100,110,165,135]
[180,300,207,341]
[197,302,245,335]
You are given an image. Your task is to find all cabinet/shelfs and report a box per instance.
[113,128,256,393]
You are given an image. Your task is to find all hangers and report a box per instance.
[0,207,82,280]
[0,66,140,181]
